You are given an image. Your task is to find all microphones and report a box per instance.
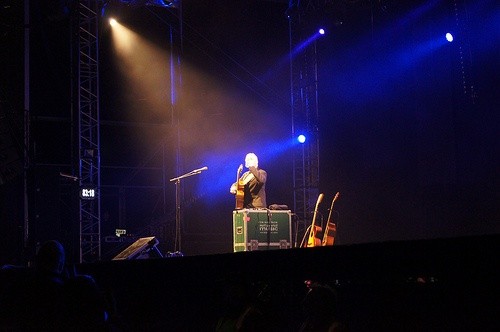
[194,166,208,171]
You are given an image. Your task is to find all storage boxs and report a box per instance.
[232,210,293,253]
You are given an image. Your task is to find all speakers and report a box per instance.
[112,236,166,261]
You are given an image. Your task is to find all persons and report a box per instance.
[230,153,267,210]
[3,241,120,331]
[216,279,271,332]
[297,284,344,331]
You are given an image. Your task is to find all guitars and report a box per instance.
[306,193,324,248]
[235,165,245,209]
[322,192,340,247]
